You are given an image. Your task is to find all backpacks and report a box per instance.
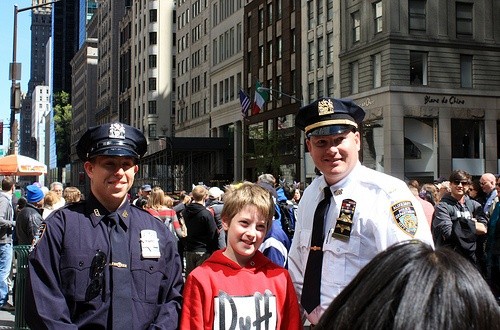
[284,203,298,230]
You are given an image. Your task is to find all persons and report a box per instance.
[124,170,309,213]
[144,186,187,250]
[12,186,45,249]
[31,122,182,330]
[429,170,486,285]
[284,98,434,330]
[205,187,229,251]
[397,171,469,205]
[1,176,15,313]
[487,174,500,301]
[37,174,155,224]
[308,238,500,330]
[478,172,499,234]
[176,186,217,290]
[180,181,304,330]
[468,181,487,207]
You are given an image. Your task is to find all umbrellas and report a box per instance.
[0,153,47,188]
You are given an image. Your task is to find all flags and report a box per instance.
[240,89,251,116]
[252,79,270,116]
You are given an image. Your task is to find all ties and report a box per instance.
[300,188,332,315]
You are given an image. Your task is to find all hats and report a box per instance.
[142,185,152,191]
[295,97,365,139]
[208,187,224,199]
[26,185,44,203]
[76,123,148,162]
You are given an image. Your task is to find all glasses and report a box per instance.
[86,250,107,298]
[451,180,468,185]
[495,183,500,187]
[468,188,475,191]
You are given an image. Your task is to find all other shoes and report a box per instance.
[2,302,15,311]
